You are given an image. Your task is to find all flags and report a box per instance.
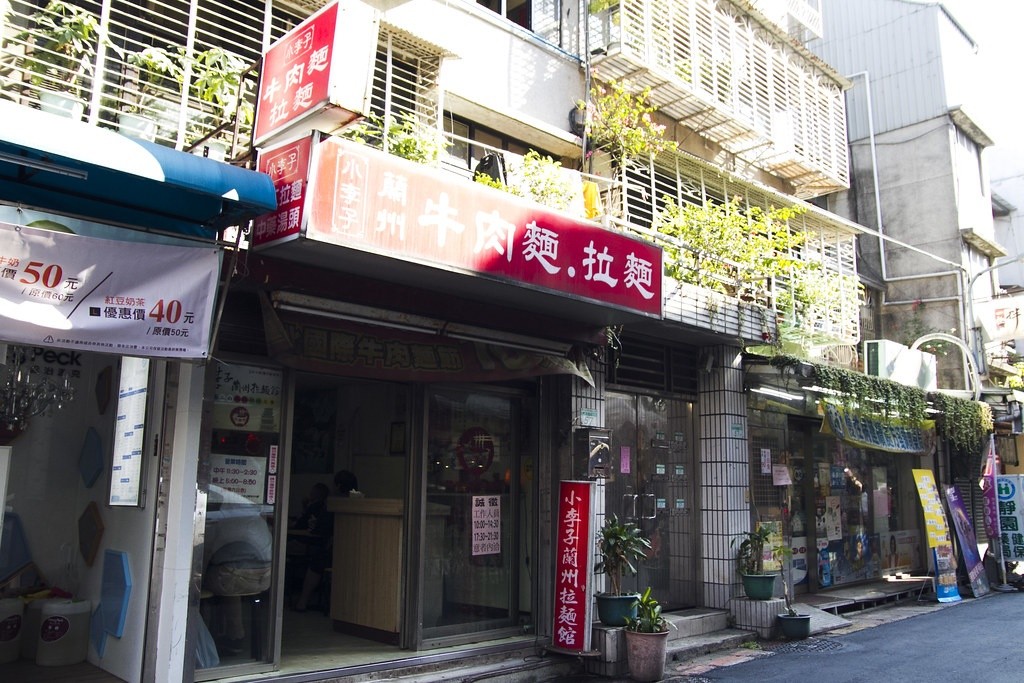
[983,446,998,536]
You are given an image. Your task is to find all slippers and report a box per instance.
[290,605,306,615]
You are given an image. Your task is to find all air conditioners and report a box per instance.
[864,339,938,390]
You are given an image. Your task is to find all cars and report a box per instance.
[202,485,271,599]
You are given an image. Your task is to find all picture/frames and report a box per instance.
[389,421,406,455]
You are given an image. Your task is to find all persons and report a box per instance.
[201,482,274,652]
[289,471,359,613]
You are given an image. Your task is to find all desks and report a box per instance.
[287,529,325,578]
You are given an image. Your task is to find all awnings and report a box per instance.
[1,98,278,362]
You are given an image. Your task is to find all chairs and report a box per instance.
[323,568,332,618]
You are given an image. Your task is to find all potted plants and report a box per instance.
[593,512,652,626]
[622,586,678,683]
[732,518,781,600]
[772,545,813,641]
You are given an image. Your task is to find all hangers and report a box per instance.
[482,145,492,164]
